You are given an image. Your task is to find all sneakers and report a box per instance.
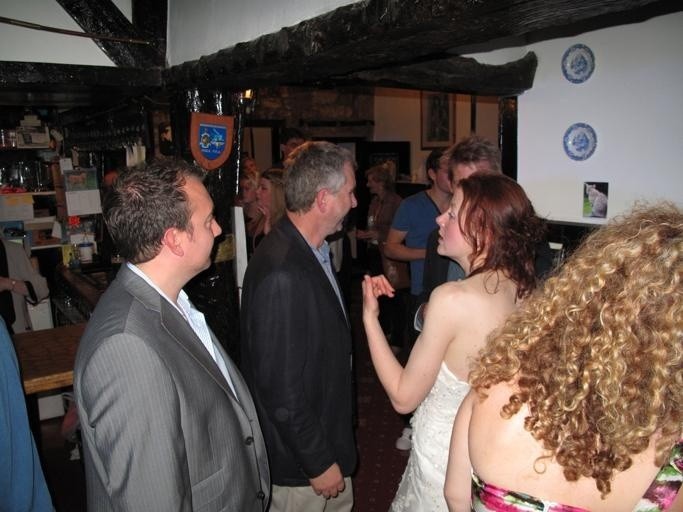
[395,426,413,450]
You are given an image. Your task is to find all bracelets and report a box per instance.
[12,280,15,292]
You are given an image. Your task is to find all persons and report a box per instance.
[0,318,54,511]
[0,227,49,457]
[74,126,683,512]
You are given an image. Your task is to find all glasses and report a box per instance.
[284,142,299,149]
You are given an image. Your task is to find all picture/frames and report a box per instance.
[420,91,456,151]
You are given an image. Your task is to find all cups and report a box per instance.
[75,243,94,265]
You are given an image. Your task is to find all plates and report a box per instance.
[563,123,597,161]
[561,43,595,83]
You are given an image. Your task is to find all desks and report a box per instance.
[10,322,85,487]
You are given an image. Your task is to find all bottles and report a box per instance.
[111,247,122,279]
